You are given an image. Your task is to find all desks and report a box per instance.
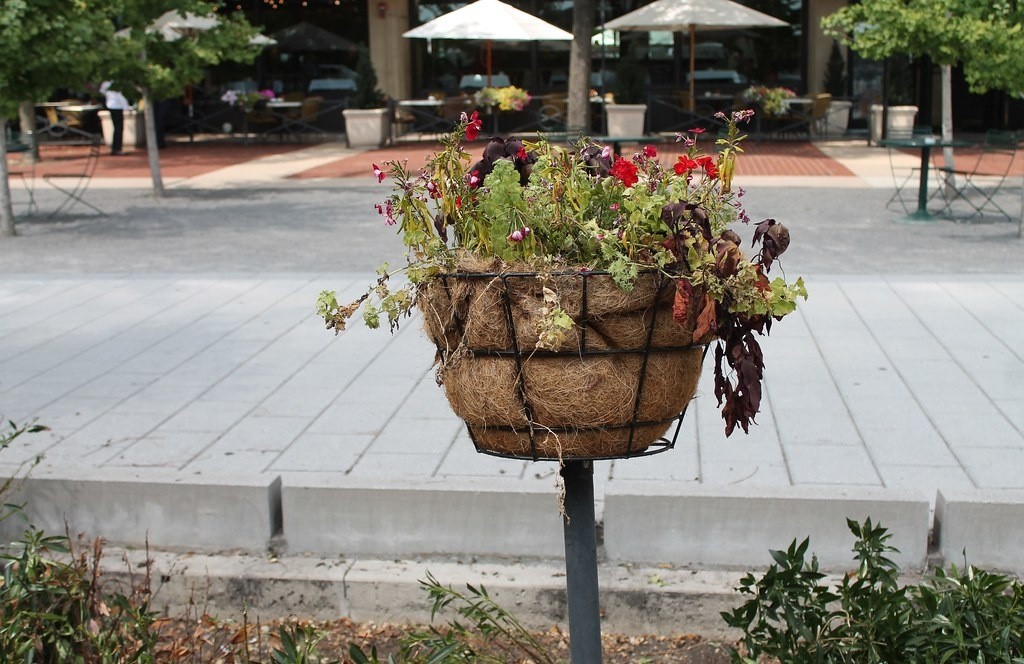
[262,101,301,140]
[883,138,972,221]
[400,99,454,129]
[36,102,102,143]
[5,143,30,216]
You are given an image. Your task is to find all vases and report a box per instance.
[96,109,145,151]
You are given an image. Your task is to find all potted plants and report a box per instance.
[810,37,853,139]
[605,57,648,147]
[870,85,920,145]
[343,43,396,150]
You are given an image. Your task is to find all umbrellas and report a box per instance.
[595,0,790,110]
[269,23,359,51]
[113,8,277,46]
[402,0,575,88]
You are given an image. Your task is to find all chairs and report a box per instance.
[764,94,833,141]
[883,125,964,220]
[936,129,1021,224]
[246,94,324,143]
[4,131,39,220]
[41,134,103,222]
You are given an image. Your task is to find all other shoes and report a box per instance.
[112,151,128,155]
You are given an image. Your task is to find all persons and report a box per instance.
[100,80,130,154]
[728,36,771,87]
[196,55,318,100]
[616,55,653,105]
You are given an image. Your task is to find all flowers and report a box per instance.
[743,85,798,118]
[221,89,278,113]
[473,85,532,112]
[316,107,809,459]
